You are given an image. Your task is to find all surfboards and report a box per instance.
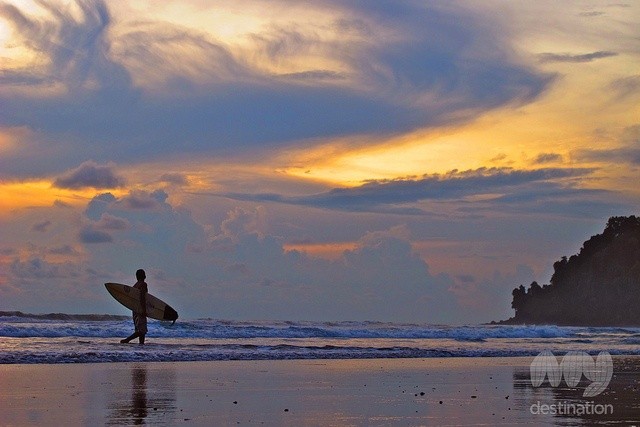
[105,283,179,321]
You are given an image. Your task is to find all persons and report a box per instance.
[120,269,148,344]
